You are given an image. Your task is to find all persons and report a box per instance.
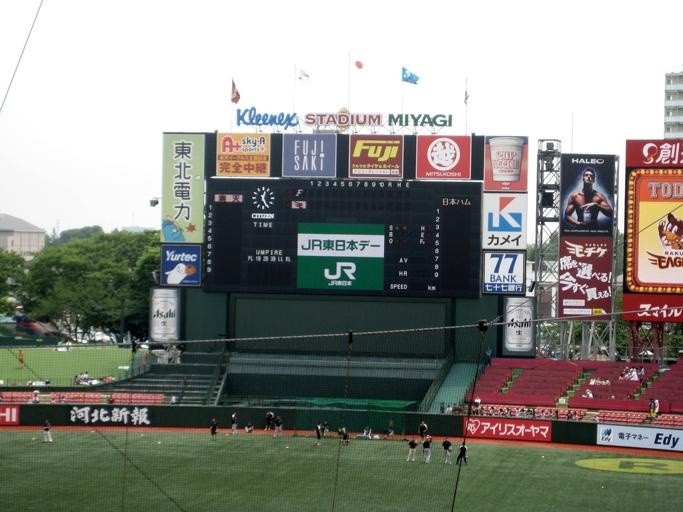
[562,167,614,227]
[209,408,472,468]
[0,339,186,406]
[647,398,656,418]
[483,346,494,367]
[580,364,646,399]
[436,393,602,424]
[41,418,52,443]
[654,399,659,414]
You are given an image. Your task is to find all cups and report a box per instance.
[488,137,525,182]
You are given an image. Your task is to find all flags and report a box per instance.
[229,79,240,104]
[401,67,419,86]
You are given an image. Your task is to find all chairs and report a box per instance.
[0,389,165,407]
[465,356,683,432]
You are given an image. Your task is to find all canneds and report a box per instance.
[150,289,179,342]
[504,297,534,352]
